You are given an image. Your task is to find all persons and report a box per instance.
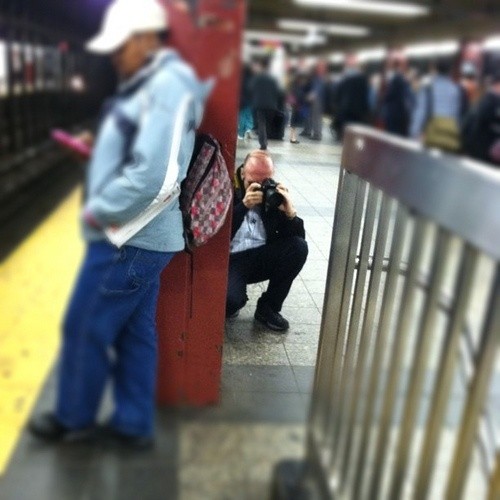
[25,0,205,453]
[225,150,309,331]
[235,41,500,166]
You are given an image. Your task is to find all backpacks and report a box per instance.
[178,132,234,319]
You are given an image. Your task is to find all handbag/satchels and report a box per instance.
[424,114,463,151]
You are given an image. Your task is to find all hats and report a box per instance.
[82,0,170,54]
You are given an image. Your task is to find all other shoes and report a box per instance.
[231,310,242,318]
[27,410,95,443]
[254,304,290,332]
[94,418,156,450]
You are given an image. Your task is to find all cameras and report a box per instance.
[253,178,283,210]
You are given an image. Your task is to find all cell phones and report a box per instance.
[52,129,90,156]
